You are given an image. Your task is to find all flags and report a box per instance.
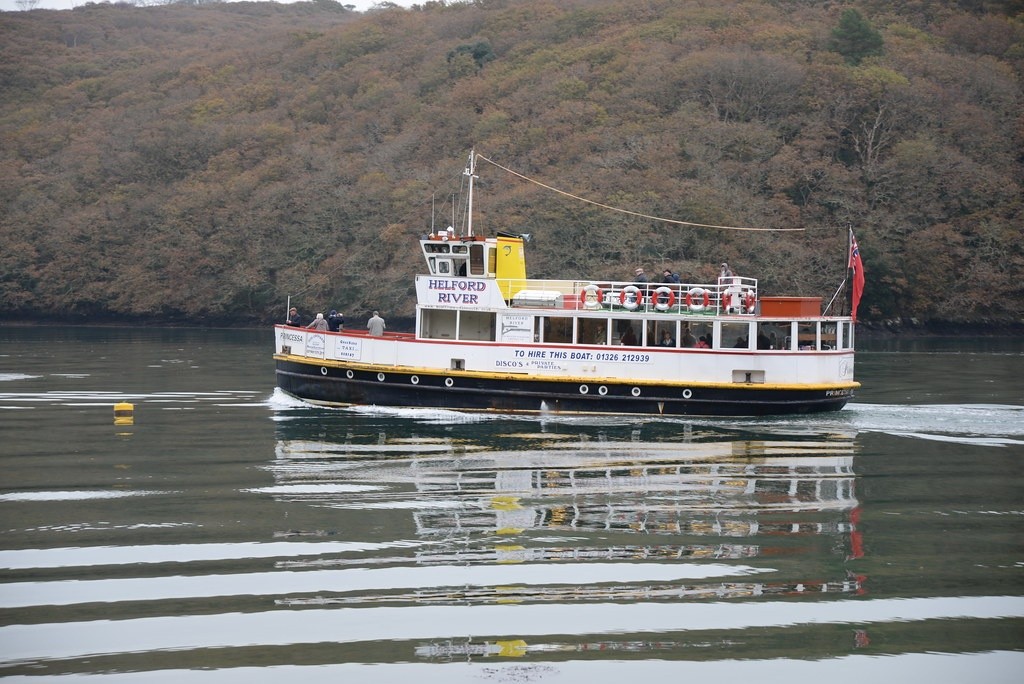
[849,234,865,322]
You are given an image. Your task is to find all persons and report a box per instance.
[366,311,386,336]
[286,307,300,327]
[594,323,606,345]
[621,326,637,345]
[327,310,344,332]
[720,262,733,291]
[624,268,647,311]
[305,313,329,331]
[757,330,770,350]
[654,270,677,311]
[638,324,655,345]
[692,333,712,348]
[733,334,748,348]
[661,330,676,347]
[668,268,681,297]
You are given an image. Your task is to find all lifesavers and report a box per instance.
[651,287,676,311]
[745,289,757,312]
[703,290,715,311]
[722,290,732,311]
[685,287,708,310]
[619,285,642,311]
[581,284,603,307]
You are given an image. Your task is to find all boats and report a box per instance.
[272,145,866,414]
[245,396,865,654]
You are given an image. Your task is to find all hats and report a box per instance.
[331,310,336,315]
[289,307,297,311]
[663,269,671,273]
[635,269,643,272]
